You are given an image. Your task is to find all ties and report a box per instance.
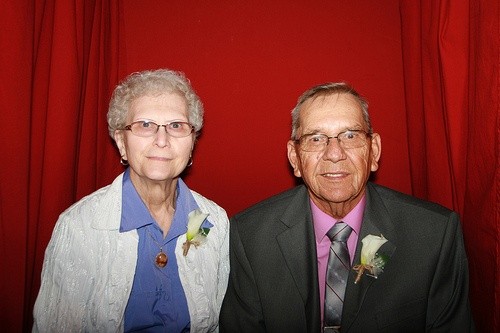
[324,222,352,333]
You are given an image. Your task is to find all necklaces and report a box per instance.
[141,226,172,269]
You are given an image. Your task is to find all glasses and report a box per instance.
[295,130,372,152]
[118,121,196,138]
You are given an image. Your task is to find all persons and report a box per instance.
[218,80,473,333]
[33,68,229,333]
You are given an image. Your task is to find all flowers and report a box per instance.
[353,235,391,285]
[182,210,214,256]
